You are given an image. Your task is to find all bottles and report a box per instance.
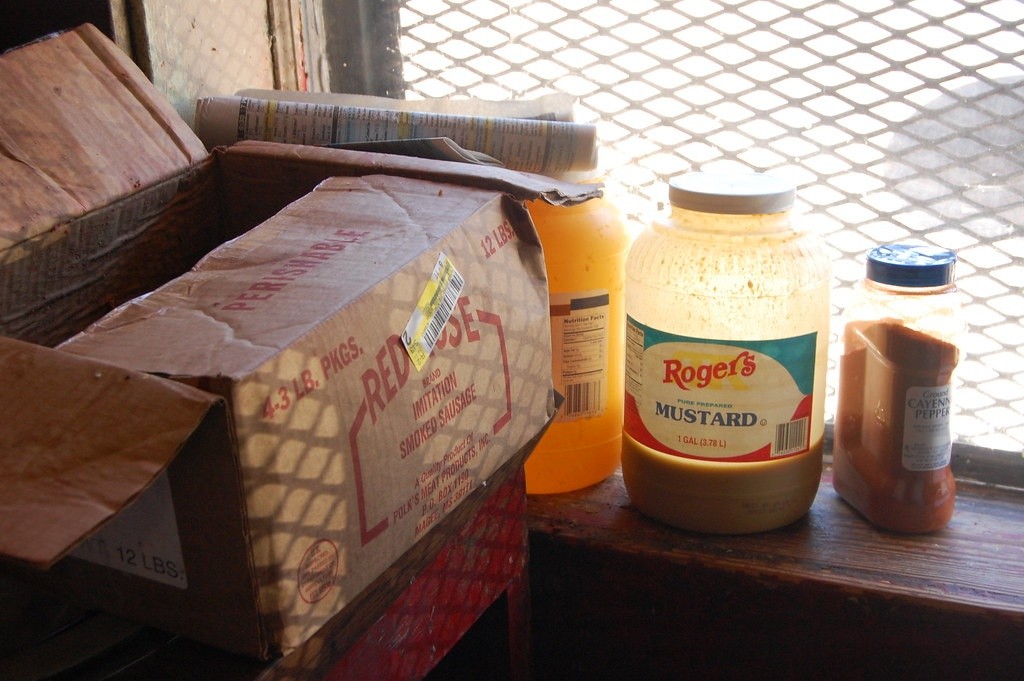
[520,184,629,496]
[620,176,827,533]
[834,241,962,533]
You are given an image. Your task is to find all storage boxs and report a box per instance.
[0,23,601,663]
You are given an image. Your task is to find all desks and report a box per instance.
[532,449,1024,627]
[251,416,580,681]
